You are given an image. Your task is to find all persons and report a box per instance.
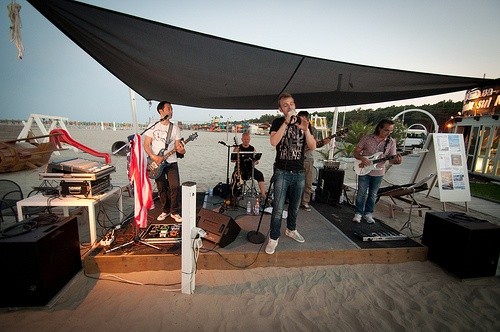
[352,119,401,224]
[265,91,315,255]
[144,101,185,222]
[232,133,268,203]
[297,111,330,212]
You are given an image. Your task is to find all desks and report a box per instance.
[16,184,124,246]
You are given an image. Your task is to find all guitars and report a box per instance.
[352,148,430,177]
[305,128,349,151]
[231,136,244,197]
[146,132,199,178]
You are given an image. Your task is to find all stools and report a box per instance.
[243,177,258,195]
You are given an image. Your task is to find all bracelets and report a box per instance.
[150,154,156,159]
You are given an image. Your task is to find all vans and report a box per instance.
[399,129,427,148]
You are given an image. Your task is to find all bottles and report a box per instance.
[247,201,251,215]
[255,199,259,215]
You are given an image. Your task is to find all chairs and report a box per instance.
[0,179,56,224]
[373,180,431,213]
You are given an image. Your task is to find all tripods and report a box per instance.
[231,152,262,200]
[398,189,417,238]
[105,121,164,254]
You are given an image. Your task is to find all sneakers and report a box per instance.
[363,214,375,224]
[353,213,362,223]
[286,228,305,243]
[265,237,279,254]
[157,212,170,221]
[302,203,311,211]
[171,213,182,222]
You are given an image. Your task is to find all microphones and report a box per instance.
[161,115,168,121]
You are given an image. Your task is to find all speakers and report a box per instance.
[0,214,81,307]
[316,170,344,205]
[198,208,242,247]
[422,211,498,276]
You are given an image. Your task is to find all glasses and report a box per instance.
[382,128,393,134]
